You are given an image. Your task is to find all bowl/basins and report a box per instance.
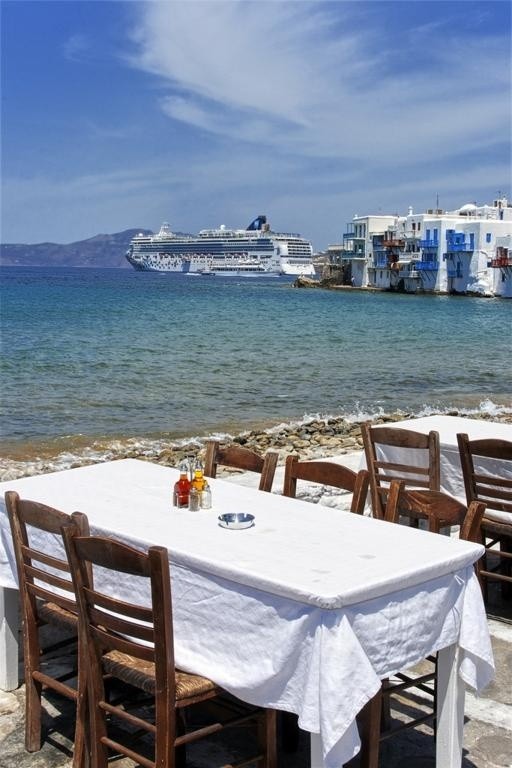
[216,512,255,530]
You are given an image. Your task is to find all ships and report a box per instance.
[125,215,317,276]
[325,193,512,299]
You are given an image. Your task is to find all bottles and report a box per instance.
[172,463,213,512]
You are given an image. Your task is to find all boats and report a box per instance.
[200,259,282,278]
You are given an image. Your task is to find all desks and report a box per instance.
[372,414,512,523]
